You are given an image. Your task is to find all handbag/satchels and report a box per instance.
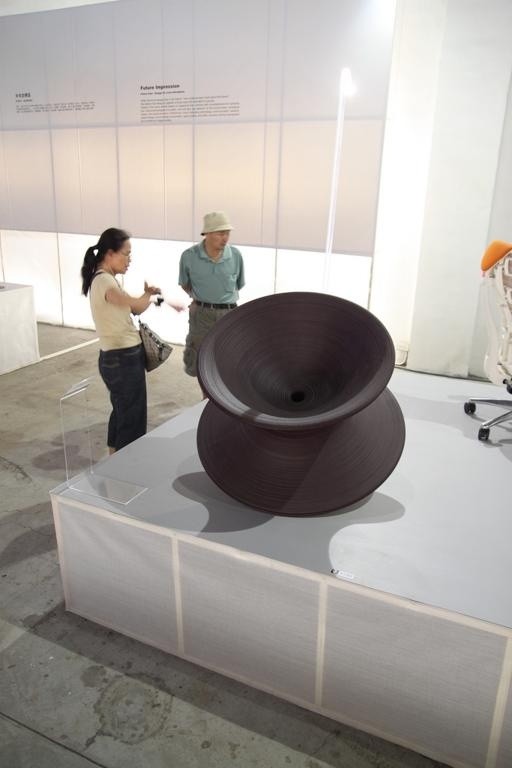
[139,323,172,372]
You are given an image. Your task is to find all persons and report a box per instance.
[177,211,245,402]
[79,226,162,454]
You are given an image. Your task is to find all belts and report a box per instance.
[195,300,237,309]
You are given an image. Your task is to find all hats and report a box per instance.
[201,212,234,235]
